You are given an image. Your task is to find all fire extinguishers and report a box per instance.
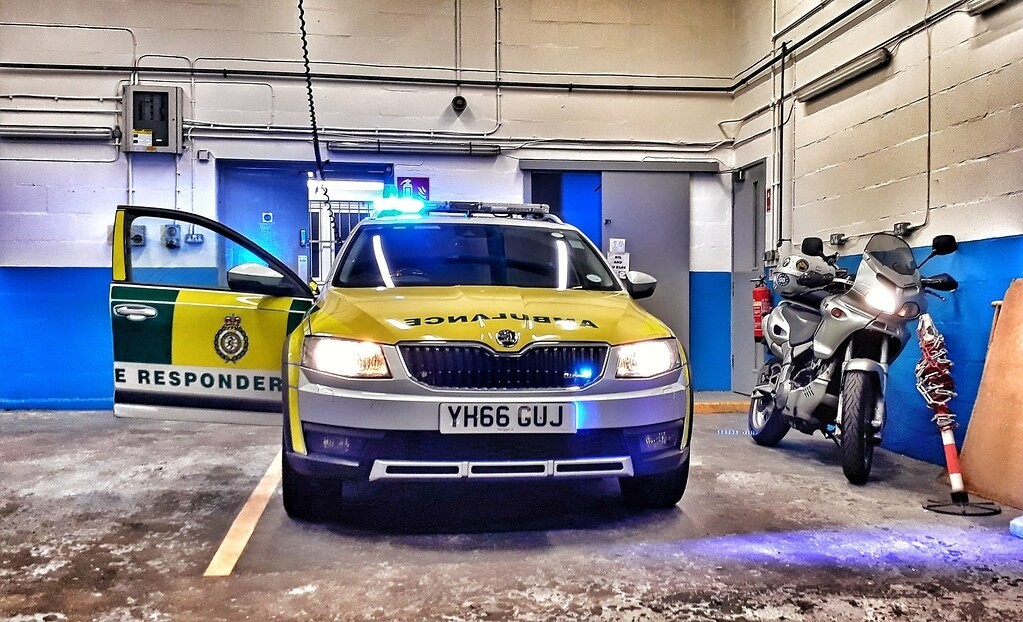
[747,274,772,345]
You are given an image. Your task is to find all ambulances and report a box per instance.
[108,204,692,516]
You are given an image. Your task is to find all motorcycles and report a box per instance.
[747,232,961,485]
